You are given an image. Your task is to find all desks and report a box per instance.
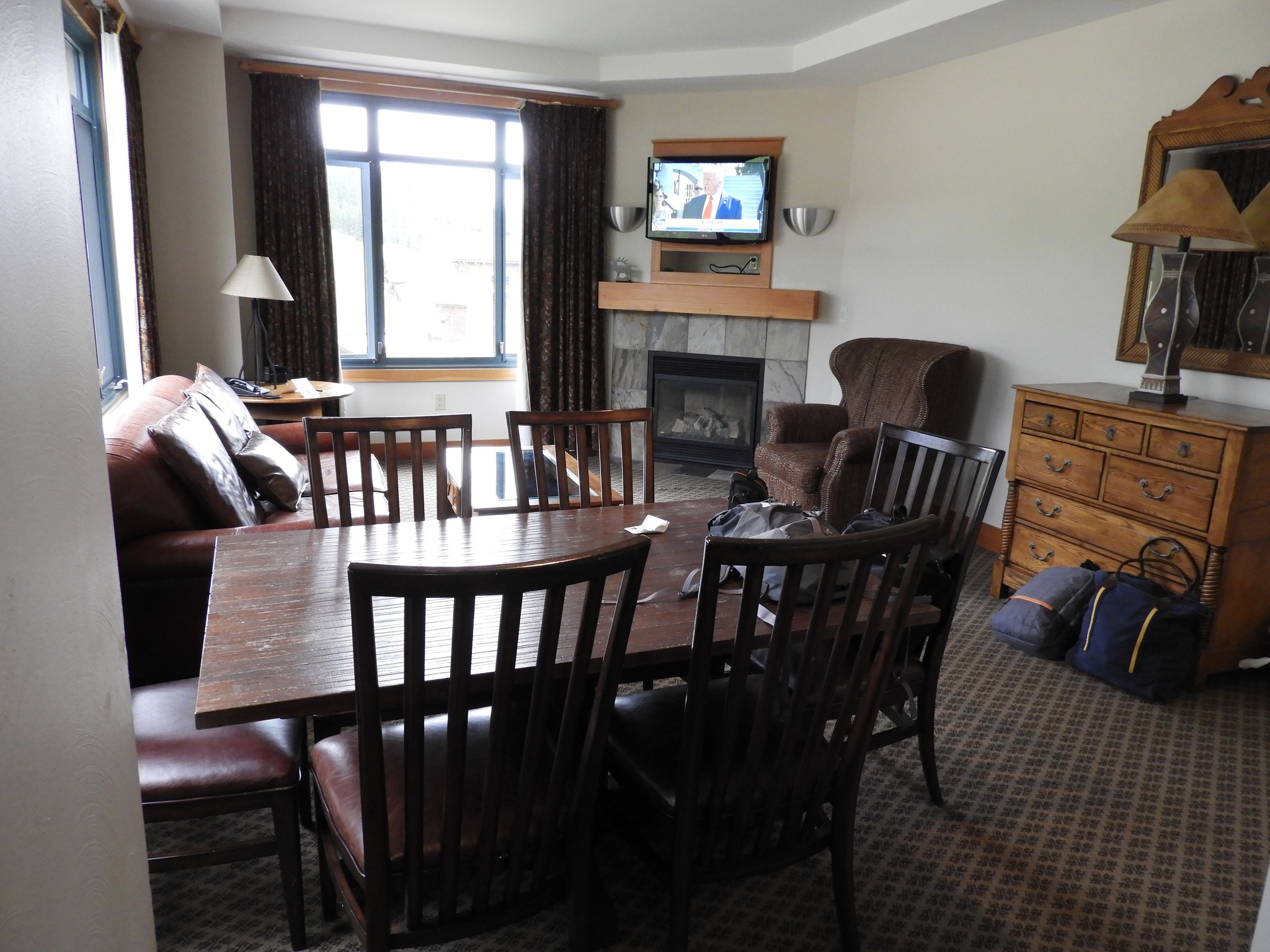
[224,380,354,422]
[192,497,940,952]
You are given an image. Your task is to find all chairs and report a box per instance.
[295,414,476,522]
[615,515,947,951]
[748,419,1004,804]
[505,407,654,514]
[754,338,972,528]
[306,533,655,952]
[133,674,311,952]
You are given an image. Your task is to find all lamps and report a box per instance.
[1236,178,1270,357]
[1108,166,1256,406]
[782,208,834,238]
[602,206,647,233]
[219,254,294,390]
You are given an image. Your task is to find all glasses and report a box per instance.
[654,195,664,198]
[694,187,703,190]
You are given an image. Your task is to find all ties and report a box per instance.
[703,196,713,219]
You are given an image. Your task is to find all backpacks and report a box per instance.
[990,560,1104,660]
[678,497,862,623]
[837,501,956,721]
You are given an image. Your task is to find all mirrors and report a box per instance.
[1114,70,1270,382]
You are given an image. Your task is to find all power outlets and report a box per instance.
[749,256,758,270]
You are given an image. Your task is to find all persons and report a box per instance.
[652,190,671,218]
[693,182,704,196]
[682,169,742,219]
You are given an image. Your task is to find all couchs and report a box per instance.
[103,374,397,616]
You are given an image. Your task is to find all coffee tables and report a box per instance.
[438,442,624,518]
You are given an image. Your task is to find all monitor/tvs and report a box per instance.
[646,155,775,245]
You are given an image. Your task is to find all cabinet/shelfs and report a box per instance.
[992,383,1270,693]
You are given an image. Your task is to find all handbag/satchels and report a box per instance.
[1064,537,1212,705]
[726,466,772,512]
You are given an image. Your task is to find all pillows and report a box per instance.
[145,365,309,527]
